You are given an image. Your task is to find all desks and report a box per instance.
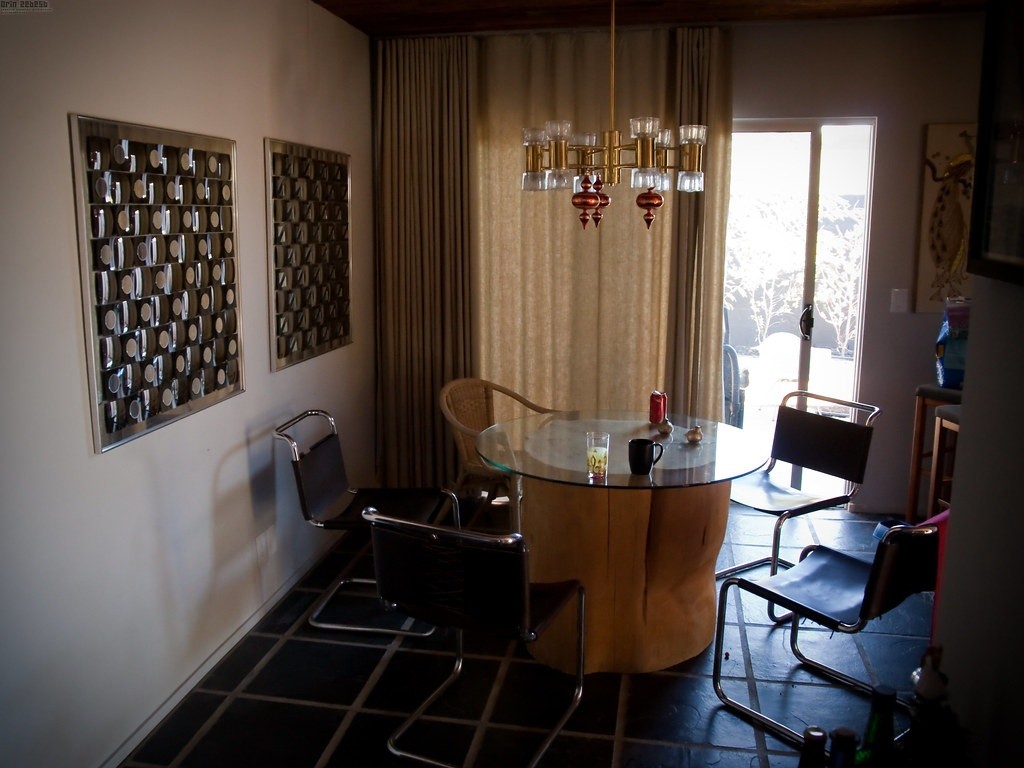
[905,384,962,525]
[476,410,774,676]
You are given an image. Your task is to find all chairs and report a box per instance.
[716,391,884,625]
[712,510,952,762]
[362,505,585,768]
[429,378,579,534]
[271,409,462,639]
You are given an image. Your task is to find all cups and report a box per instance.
[628,439,663,476]
[587,431,610,482]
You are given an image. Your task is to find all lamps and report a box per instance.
[521,0,705,230]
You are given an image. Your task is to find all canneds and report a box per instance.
[649,390,667,423]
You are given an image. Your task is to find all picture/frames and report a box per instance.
[966,0,1024,291]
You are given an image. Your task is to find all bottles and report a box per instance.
[649,391,667,422]
[658,419,674,434]
[686,425,703,442]
